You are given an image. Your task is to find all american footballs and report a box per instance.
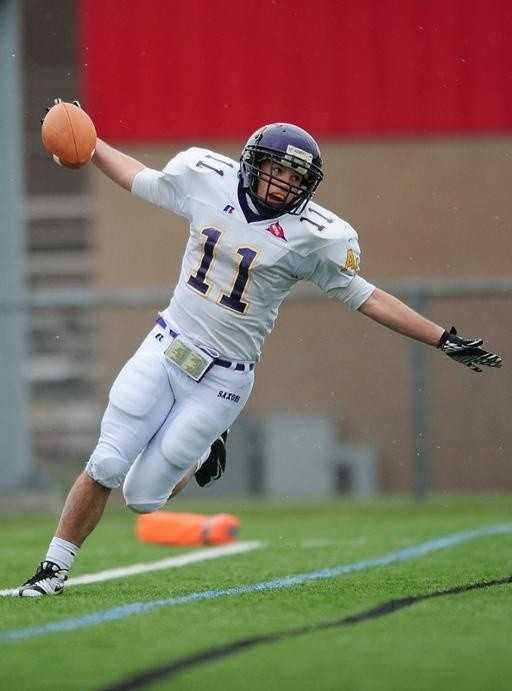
[41,102,97,168]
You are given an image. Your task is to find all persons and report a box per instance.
[19,96,504,598]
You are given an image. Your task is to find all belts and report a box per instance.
[155,314,255,373]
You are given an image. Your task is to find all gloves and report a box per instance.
[40,97,80,125]
[435,326,504,373]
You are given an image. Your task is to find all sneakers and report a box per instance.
[9,559,71,599]
[193,427,230,490]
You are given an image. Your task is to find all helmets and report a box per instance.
[238,121,325,218]
